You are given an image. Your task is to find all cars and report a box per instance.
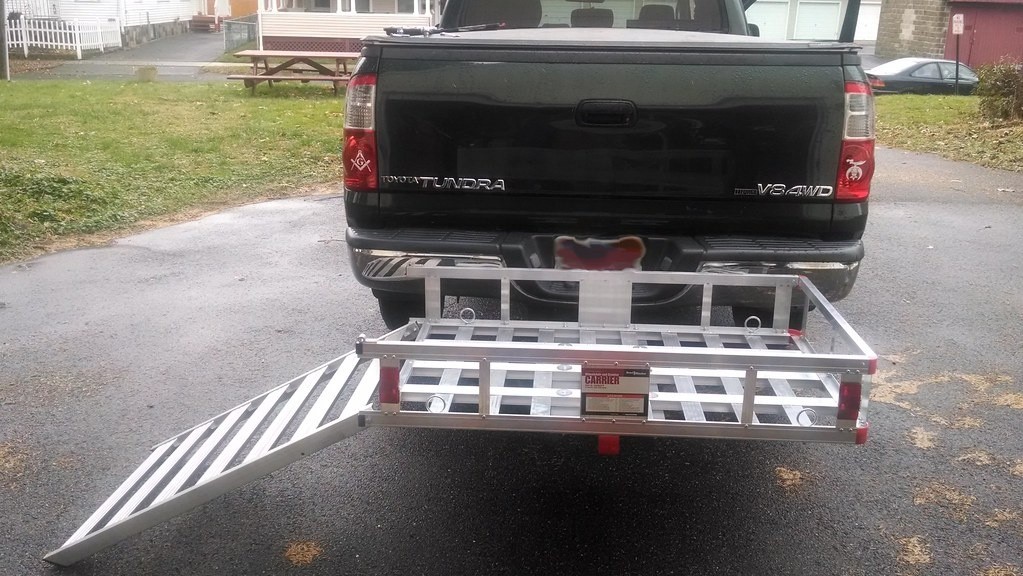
[864,57,983,96]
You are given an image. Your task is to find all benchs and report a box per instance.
[227,66,353,96]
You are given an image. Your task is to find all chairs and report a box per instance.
[571,8,614,27]
[639,4,674,19]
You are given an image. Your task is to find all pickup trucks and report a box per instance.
[341,0,876,353]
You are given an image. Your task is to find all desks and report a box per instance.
[233,50,360,96]
